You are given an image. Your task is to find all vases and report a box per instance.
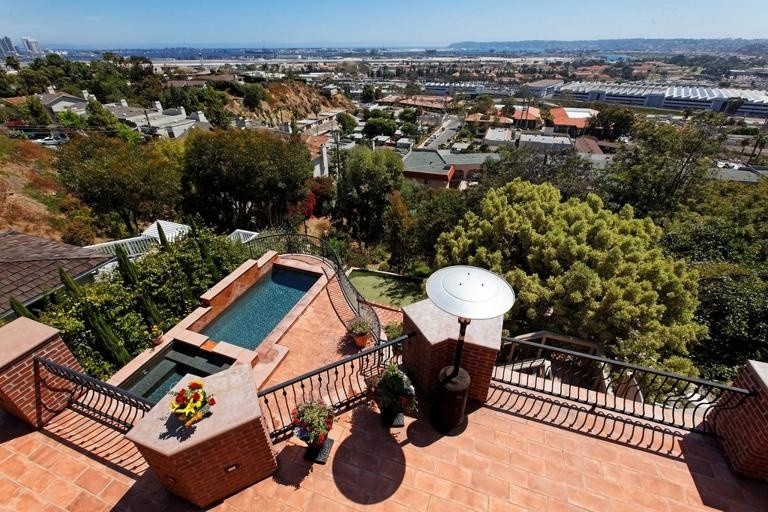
[304,407,404,462]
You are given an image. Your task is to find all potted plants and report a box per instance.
[349,316,373,348]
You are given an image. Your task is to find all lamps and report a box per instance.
[425,263,515,434]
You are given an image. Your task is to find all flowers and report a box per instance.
[290,365,416,443]
[168,380,217,428]
